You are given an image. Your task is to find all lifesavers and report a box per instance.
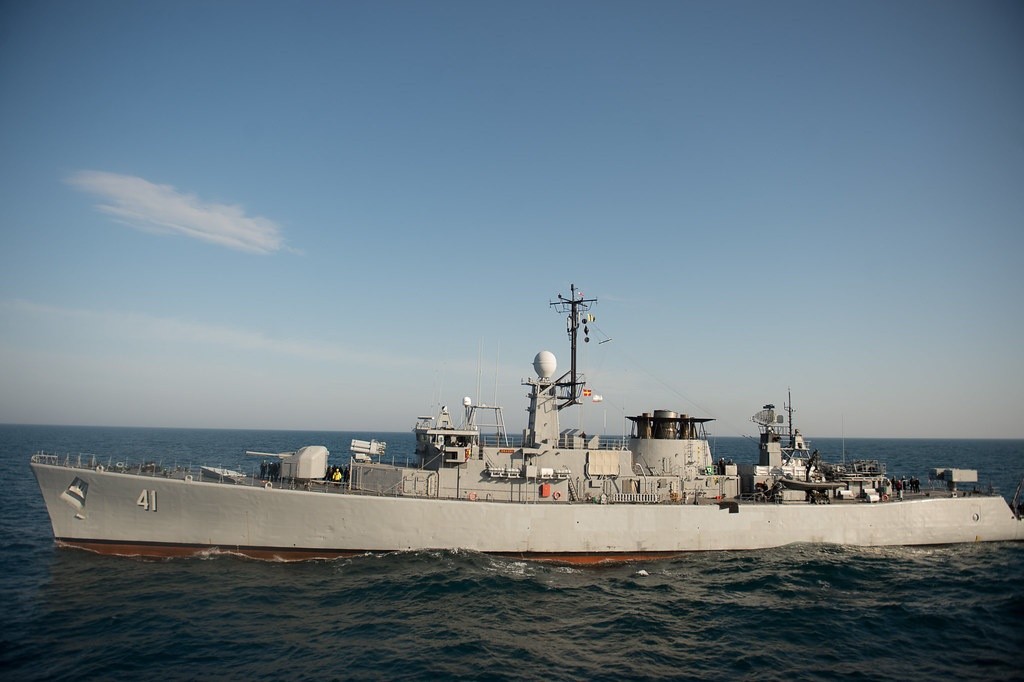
[469,491,479,501]
[882,494,889,502]
[553,490,561,500]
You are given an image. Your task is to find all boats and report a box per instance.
[31,280,1024,564]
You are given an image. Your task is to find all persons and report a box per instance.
[717,458,733,475]
[323,464,350,487]
[445,438,471,457]
[260,460,280,482]
[891,475,920,498]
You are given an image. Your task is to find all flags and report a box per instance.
[583,389,591,396]
[593,395,602,402]
